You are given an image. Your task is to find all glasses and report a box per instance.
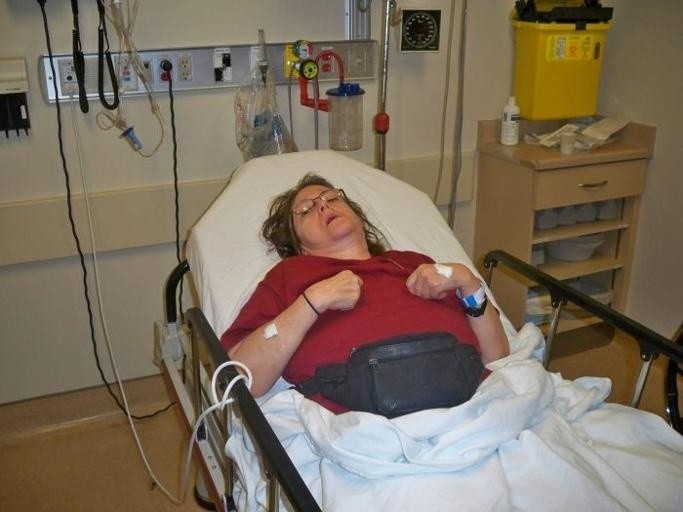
[289,188,348,214]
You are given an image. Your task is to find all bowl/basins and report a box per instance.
[546,237,604,260]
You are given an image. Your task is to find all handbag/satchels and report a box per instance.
[290,331,485,419]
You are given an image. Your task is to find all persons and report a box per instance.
[218,176,509,415]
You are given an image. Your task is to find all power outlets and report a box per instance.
[59,59,80,96]
[155,56,172,83]
[179,55,193,81]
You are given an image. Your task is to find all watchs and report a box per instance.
[460,293,488,318]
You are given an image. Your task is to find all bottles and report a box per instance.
[500,96,521,146]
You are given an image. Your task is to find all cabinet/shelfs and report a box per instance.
[476,116,655,350]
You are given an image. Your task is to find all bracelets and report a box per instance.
[302,291,319,315]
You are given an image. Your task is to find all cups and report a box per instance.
[561,132,577,154]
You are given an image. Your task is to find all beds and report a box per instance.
[155,147,681,511]
